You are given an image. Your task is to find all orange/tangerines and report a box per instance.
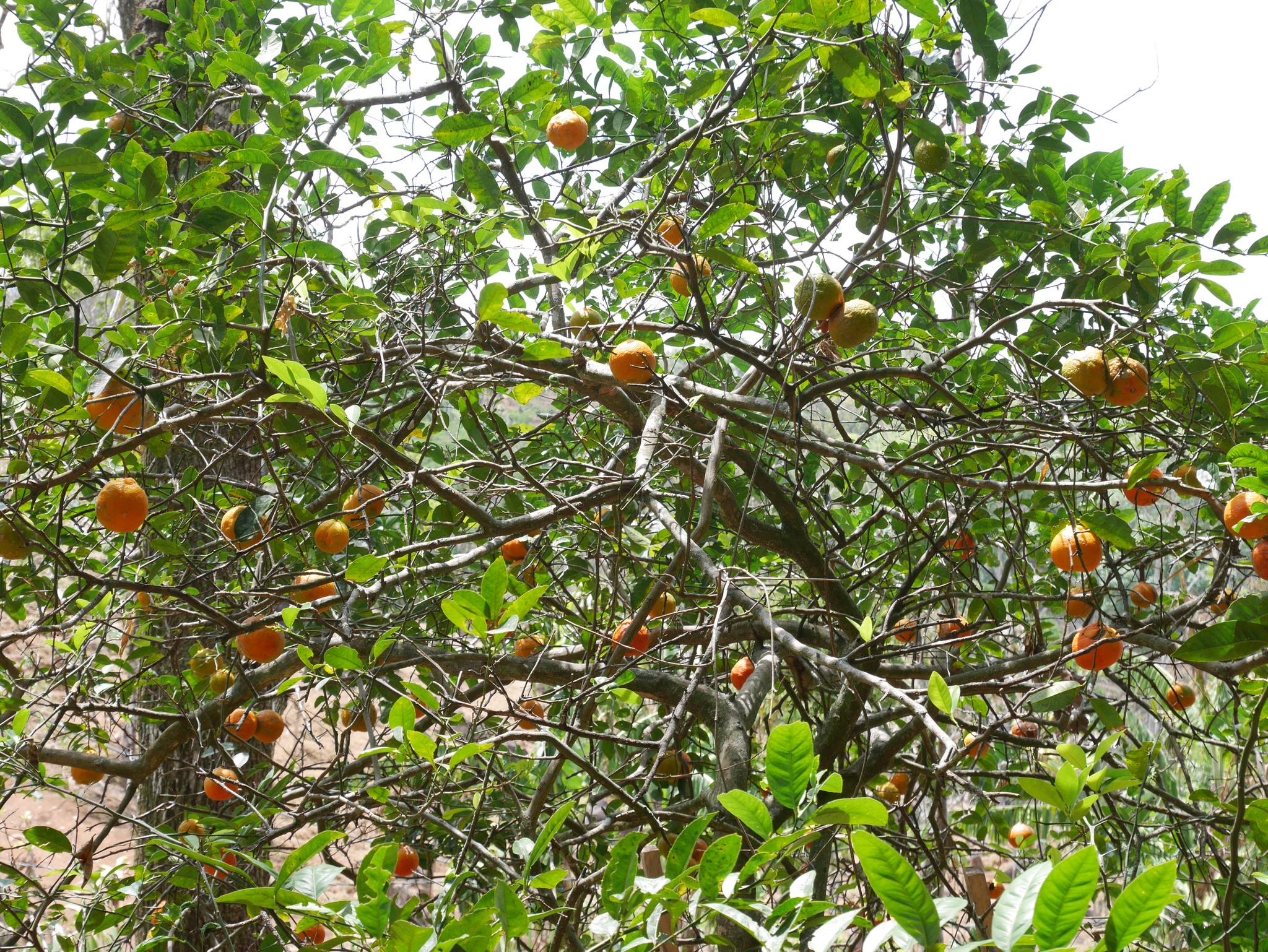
[0,112,1268,952]
